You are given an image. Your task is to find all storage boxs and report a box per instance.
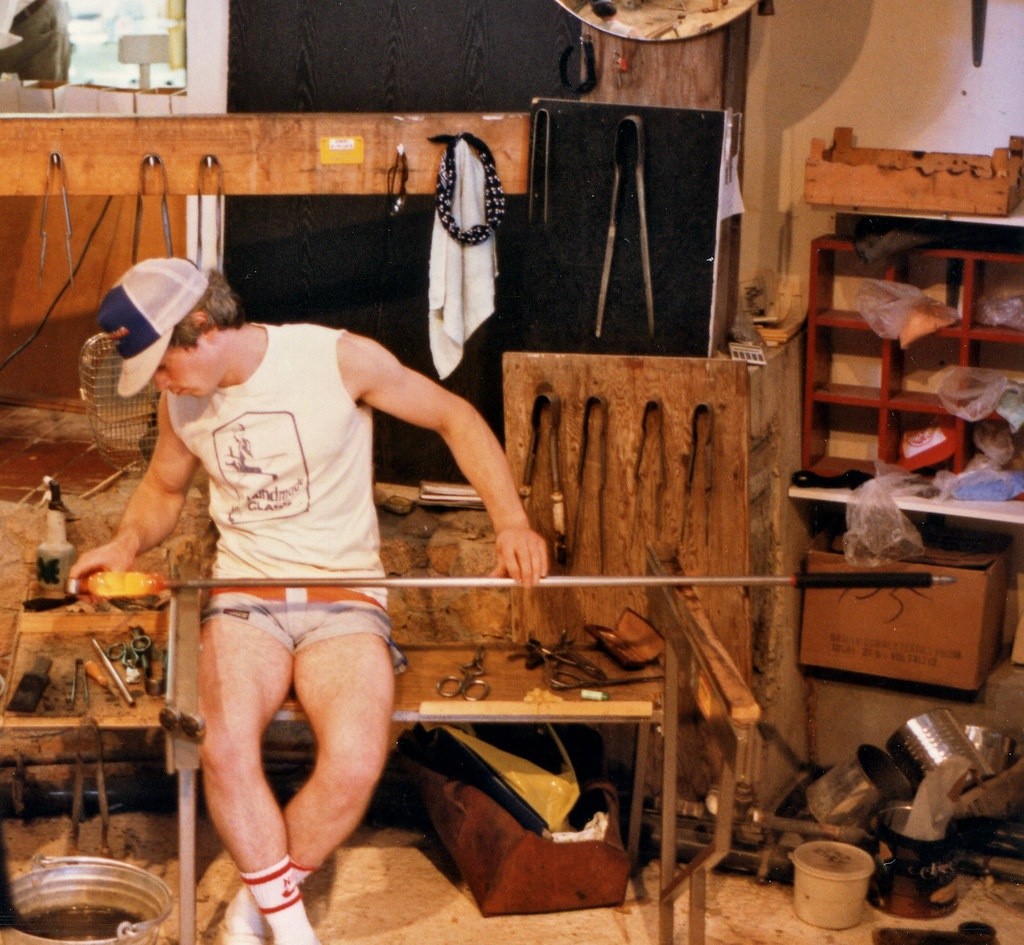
[801,120,1024,217]
[794,538,1008,692]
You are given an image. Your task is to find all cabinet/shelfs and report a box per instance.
[799,239,1024,502]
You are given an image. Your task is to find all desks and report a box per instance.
[172,631,682,945]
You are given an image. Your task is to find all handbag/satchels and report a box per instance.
[398,719,630,918]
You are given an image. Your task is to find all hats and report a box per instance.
[99,257,208,398]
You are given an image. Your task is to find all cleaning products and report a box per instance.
[36,475,76,591]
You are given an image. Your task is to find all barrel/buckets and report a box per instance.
[0,856,174,945]
[963,724,1017,776]
[872,807,963,919]
[886,707,997,797]
[806,744,911,841]
[792,841,875,929]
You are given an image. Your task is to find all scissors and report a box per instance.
[436,646,490,702]
[106,635,152,681]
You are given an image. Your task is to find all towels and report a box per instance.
[424,139,500,382]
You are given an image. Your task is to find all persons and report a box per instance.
[67,256,551,944]
